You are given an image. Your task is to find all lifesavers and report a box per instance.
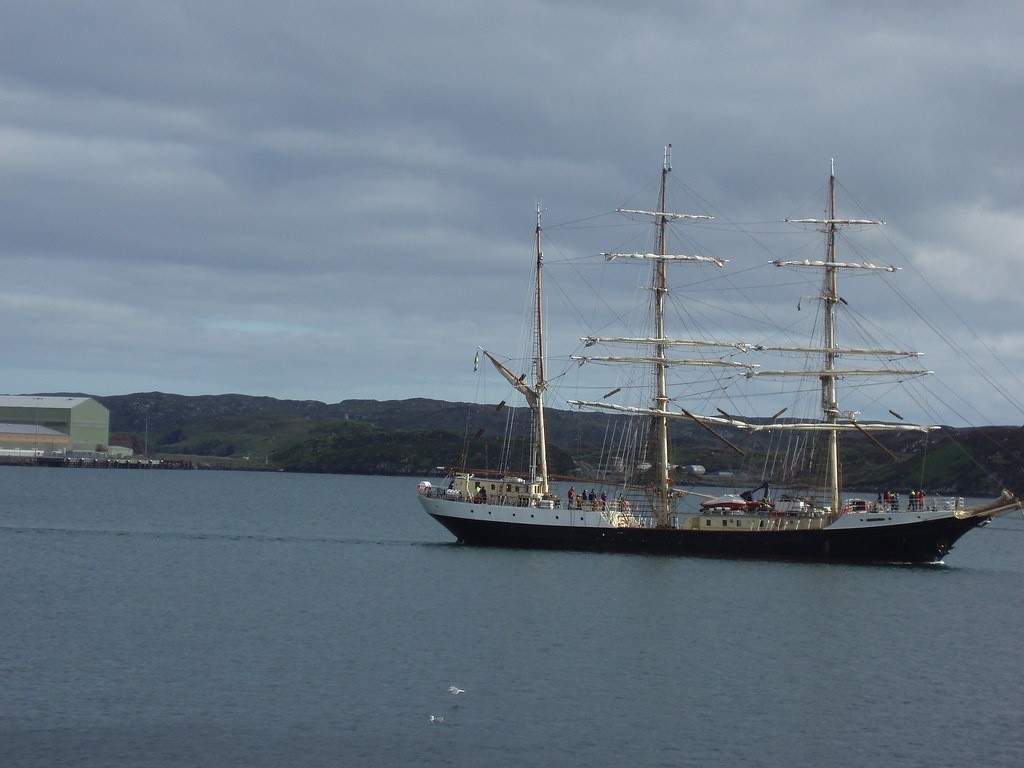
[840,504,852,516]
[424,485,430,494]
[624,501,629,509]
[775,518,784,527]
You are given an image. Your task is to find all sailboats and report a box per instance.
[416,148,1024,564]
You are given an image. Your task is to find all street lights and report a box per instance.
[32,397,43,465]
[142,404,149,459]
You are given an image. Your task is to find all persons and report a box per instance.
[582,489,587,500]
[479,486,486,503]
[878,490,899,509]
[618,493,624,511]
[589,489,597,502]
[600,490,607,500]
[908,489,926,510]
[568,487,575,510]
